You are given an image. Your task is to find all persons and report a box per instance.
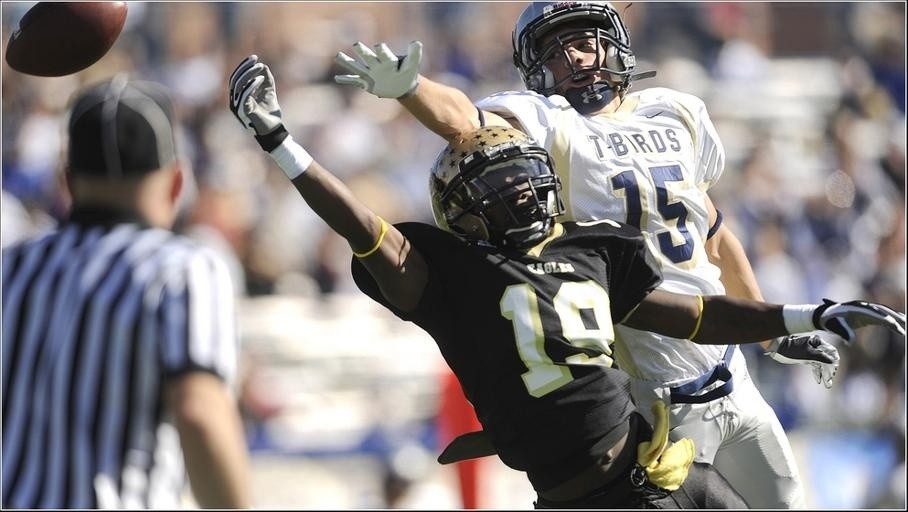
[0,1,906,510]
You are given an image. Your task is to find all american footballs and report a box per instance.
[6,2,130,78]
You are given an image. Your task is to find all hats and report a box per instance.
[66,74,175,177]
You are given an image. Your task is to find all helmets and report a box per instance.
[511,2,657,114]
[429,125,567,250]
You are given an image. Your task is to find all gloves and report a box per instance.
[764,334,840,389]
[813,298,906,344]
[333,40,422,100]
[227,54,289,153]
